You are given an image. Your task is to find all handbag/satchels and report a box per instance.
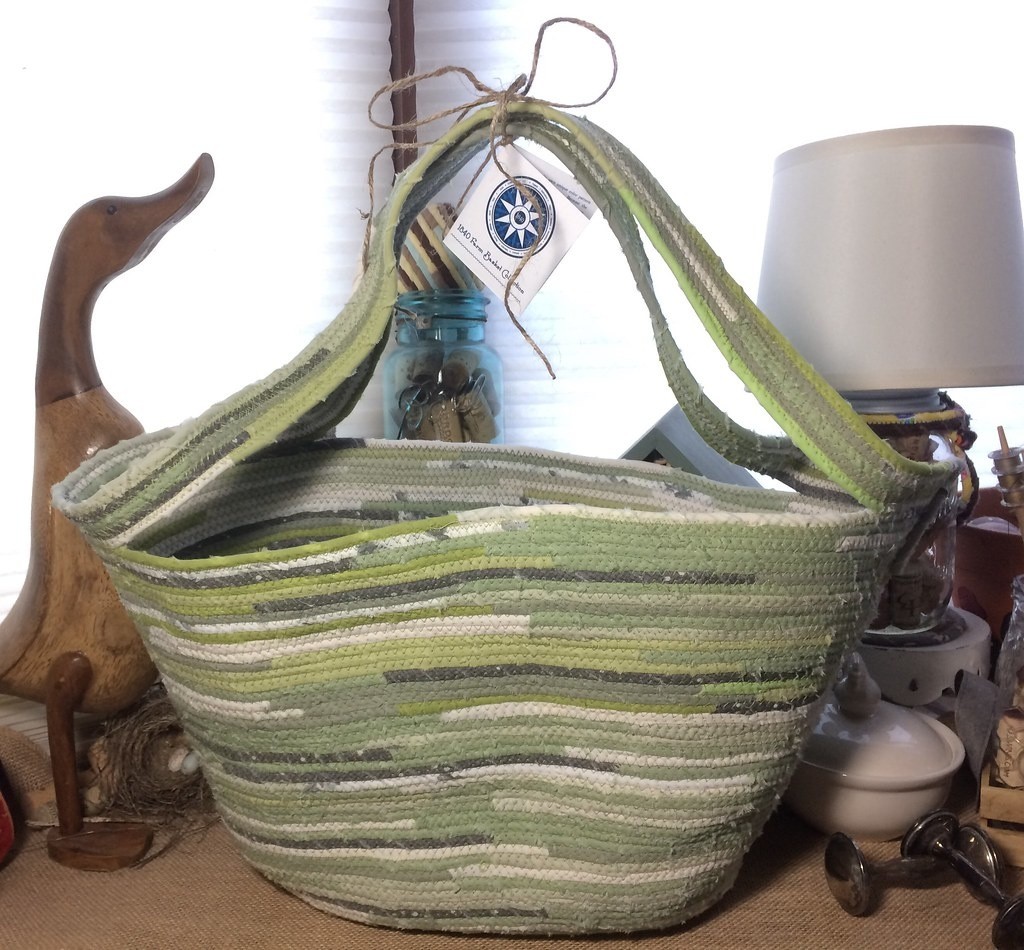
[50,101,966,932]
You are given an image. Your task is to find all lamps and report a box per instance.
[745,124,1024,649]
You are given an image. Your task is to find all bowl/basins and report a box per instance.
[787,663,967,842]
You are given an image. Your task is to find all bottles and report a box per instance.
[383,288,505,446]
